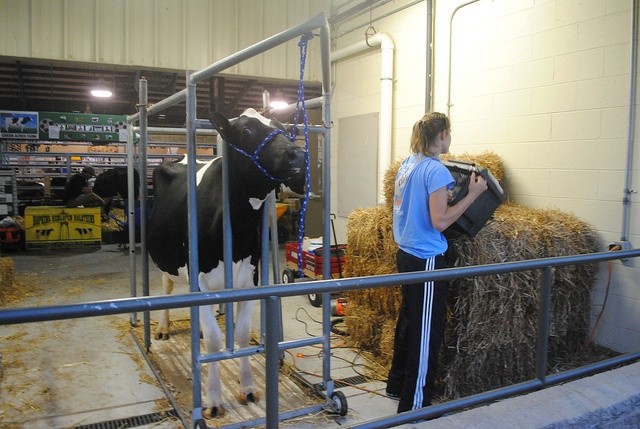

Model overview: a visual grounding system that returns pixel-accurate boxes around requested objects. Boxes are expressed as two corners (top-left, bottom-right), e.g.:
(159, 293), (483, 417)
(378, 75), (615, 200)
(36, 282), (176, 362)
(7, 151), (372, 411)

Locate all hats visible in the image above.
(83, 166), (96, 175)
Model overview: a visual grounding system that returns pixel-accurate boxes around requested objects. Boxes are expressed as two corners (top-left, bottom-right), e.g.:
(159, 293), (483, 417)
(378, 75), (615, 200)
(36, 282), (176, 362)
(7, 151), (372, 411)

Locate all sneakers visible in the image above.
(387, 391), (401, 400)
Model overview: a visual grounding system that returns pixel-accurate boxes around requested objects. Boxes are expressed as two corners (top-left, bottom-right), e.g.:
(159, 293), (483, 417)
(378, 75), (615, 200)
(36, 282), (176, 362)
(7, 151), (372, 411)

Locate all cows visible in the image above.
(147, 107), (308, 418)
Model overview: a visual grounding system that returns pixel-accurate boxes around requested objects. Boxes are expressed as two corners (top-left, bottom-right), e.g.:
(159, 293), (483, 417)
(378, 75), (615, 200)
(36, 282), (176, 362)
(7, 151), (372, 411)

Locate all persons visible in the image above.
(386, 112), (488, 413)
(64, 167), (108, 208)
